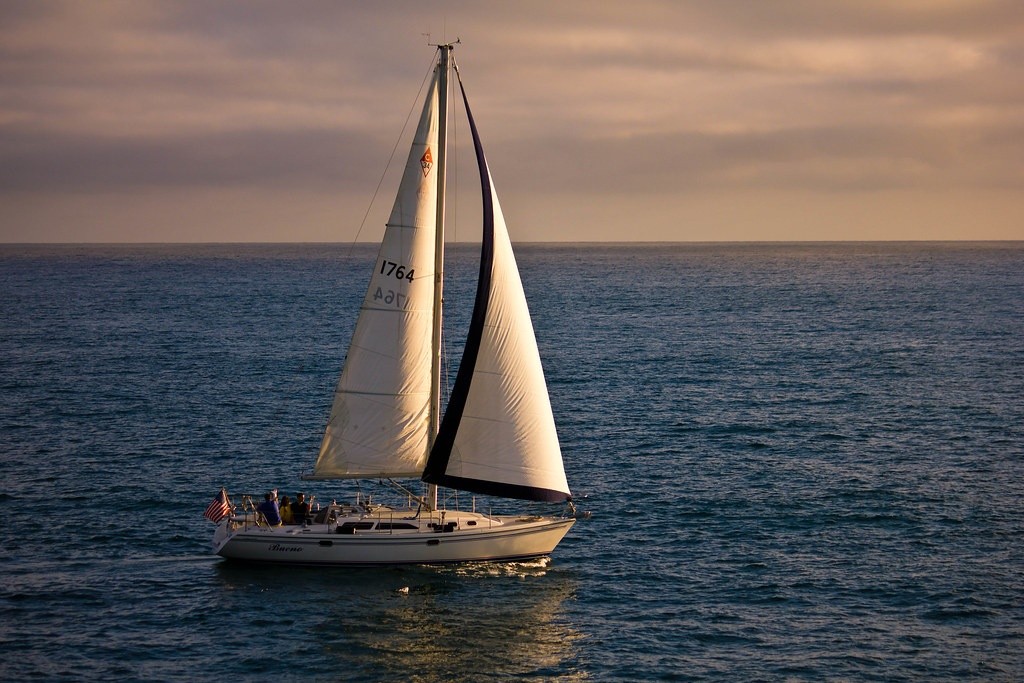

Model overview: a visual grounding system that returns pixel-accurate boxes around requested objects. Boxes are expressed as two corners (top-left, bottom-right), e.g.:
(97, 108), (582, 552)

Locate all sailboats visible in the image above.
(209, 32), (577, 565)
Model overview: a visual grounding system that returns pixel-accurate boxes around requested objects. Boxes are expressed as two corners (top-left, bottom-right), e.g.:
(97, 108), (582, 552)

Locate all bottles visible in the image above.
(316, 502), (320, 511)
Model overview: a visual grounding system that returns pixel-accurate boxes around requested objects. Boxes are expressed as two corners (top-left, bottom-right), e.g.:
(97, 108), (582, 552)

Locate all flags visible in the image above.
(203, 489), (232, 524)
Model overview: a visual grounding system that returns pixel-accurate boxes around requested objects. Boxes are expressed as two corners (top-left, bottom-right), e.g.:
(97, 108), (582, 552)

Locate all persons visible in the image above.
(257, 494), (282, 527)
(290, 493), (309, 524)
(279, 496), (292, 523)
